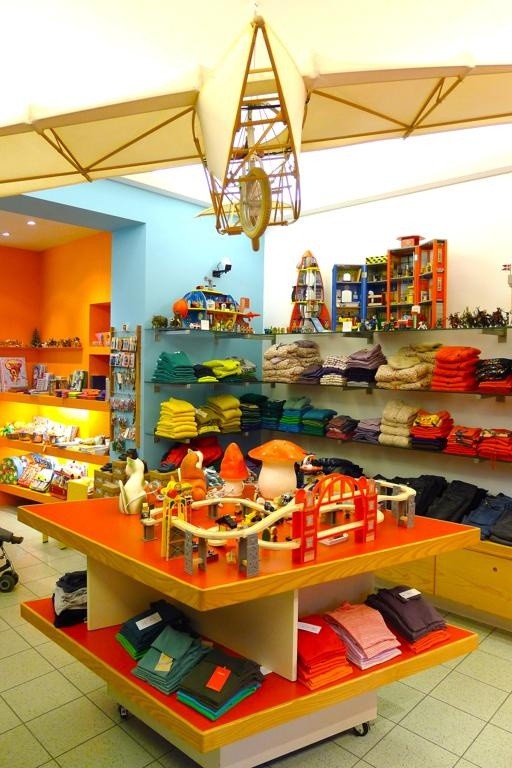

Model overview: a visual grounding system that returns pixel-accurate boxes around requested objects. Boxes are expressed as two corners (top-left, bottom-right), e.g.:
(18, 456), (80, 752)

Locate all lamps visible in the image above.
(213, 257), (232, 278)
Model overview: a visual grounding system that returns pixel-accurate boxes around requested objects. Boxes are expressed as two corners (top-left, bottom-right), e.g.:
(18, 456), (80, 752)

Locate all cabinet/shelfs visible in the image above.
(1, 324), (511, 768)
